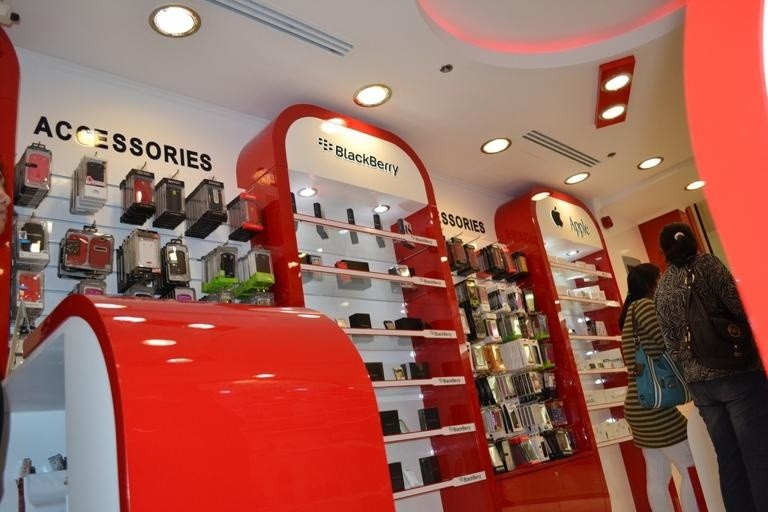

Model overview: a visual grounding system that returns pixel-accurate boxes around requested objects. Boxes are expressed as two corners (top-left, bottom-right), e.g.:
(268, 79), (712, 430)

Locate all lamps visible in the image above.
(299, 186), (317, 198)
(480, 138), (511, 155)
(600, 105), (625, 120)
(353, 85), (392, 108)
(686, 180), (704, 190)
(638, 157), (663, 171)
(601, 74), (631, 92)
(568, 249), (579, 258)
(563, 171), (589, 185)
(372, 205), (390, 215)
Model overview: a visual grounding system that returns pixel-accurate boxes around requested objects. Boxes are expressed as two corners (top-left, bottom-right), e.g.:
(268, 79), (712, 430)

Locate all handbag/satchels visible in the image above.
(636, 337), (695, 410)
(684, 281), (759, 371)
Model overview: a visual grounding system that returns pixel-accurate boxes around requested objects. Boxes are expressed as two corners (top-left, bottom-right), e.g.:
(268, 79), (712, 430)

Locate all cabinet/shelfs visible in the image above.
(495, 188), (708, 512)
(444, 227), (607, 510)
(240, 104), (494, 511)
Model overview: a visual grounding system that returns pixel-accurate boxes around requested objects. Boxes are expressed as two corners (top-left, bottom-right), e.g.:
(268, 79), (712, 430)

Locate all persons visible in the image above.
(652, 220), (767, 512)
(618, 260), (705, 511)
